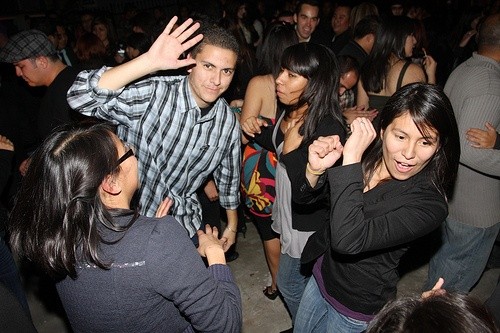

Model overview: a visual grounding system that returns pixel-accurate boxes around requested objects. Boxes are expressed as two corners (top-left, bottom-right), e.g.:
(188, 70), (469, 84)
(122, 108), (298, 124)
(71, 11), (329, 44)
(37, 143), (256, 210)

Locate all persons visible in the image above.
(292, 82), (462, 333)
(7, 119), (243, 333)
(0, 0), (500, 333)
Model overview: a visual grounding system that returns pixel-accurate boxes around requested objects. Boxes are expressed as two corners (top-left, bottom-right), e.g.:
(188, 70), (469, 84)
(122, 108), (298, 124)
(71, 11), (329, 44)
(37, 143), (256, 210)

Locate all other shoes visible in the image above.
(225, 251), (239, 262)
(280, 328), (293, 333)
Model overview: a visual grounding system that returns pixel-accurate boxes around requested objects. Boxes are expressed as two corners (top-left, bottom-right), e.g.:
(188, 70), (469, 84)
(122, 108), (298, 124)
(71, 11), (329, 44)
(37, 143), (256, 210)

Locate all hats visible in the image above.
(0, 30), (54, 63)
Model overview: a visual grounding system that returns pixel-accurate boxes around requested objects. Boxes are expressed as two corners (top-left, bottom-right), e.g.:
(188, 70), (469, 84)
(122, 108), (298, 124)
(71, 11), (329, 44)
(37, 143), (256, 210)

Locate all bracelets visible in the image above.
(305, 161), (326, 176)
(226, 226), (237, 233)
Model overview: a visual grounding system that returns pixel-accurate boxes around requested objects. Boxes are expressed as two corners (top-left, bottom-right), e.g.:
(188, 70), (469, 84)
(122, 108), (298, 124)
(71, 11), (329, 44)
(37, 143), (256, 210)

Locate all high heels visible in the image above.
(237, 223), (247, 238)
(263, 286), (282, 300)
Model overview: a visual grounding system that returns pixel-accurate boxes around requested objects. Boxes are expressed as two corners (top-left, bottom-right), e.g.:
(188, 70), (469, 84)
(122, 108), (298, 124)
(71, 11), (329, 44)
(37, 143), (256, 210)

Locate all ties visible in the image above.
(58, 52), (68, 66)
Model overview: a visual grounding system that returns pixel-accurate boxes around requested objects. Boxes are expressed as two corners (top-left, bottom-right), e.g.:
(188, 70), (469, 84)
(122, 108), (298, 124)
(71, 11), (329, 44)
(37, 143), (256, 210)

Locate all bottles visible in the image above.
(116, 43), (125, 57)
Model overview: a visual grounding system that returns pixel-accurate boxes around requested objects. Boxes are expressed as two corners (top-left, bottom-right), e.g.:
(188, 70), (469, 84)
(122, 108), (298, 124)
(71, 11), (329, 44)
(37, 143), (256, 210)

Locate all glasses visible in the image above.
(105, 141), (136, 176)
